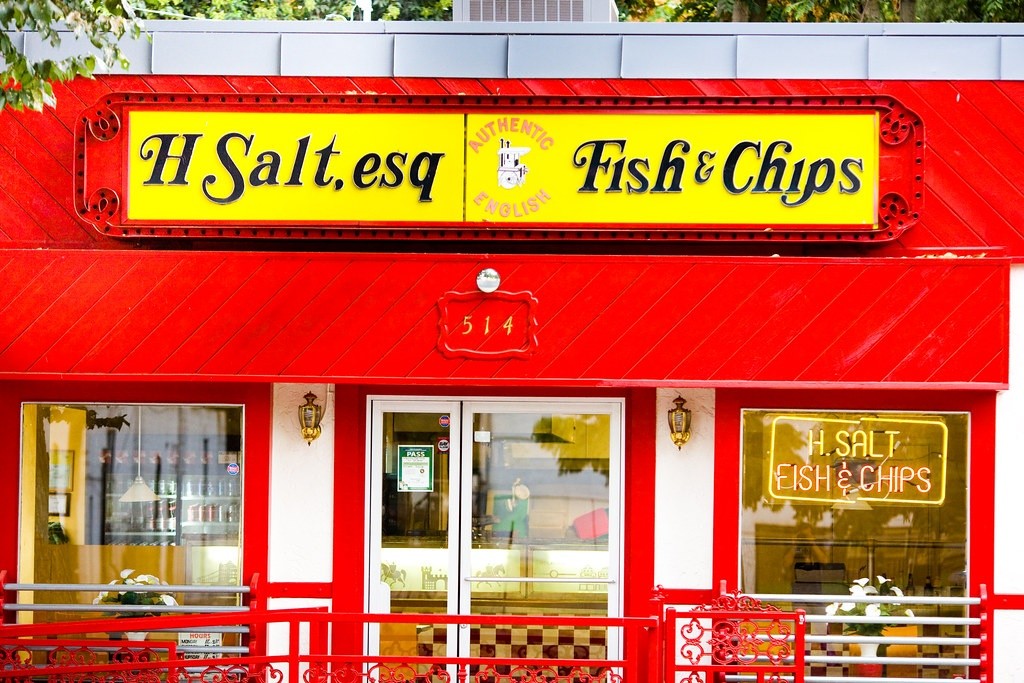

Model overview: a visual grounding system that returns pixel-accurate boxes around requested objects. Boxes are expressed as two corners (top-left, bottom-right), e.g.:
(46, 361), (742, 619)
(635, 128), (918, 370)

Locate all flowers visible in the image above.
(92, 568), (179, 619)
(824, 576), (914, 637)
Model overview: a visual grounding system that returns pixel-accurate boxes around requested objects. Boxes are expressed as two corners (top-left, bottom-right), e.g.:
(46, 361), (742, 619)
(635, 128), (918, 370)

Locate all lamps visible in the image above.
(118, 406), (161, 503)
(668, 395), (691, 451)
(298, 391), (321, 445)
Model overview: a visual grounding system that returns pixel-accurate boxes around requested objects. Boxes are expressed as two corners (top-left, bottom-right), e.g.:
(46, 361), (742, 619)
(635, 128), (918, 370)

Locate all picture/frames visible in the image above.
(49, 449), (74, 492)
(47, 492), (71, 517)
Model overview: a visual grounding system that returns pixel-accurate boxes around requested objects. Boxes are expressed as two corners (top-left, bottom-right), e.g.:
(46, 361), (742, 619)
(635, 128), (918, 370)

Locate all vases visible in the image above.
(856, 644), (883, 677)
(121, 632), (150, 641)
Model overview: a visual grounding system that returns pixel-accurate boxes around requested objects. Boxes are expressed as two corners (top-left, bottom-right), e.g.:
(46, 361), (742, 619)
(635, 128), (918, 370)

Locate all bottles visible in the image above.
(879, 573), (889, 595)
(905, 573), (915, 596)
(890, 577), (897, 596)
(924, 576), (933, 596)
